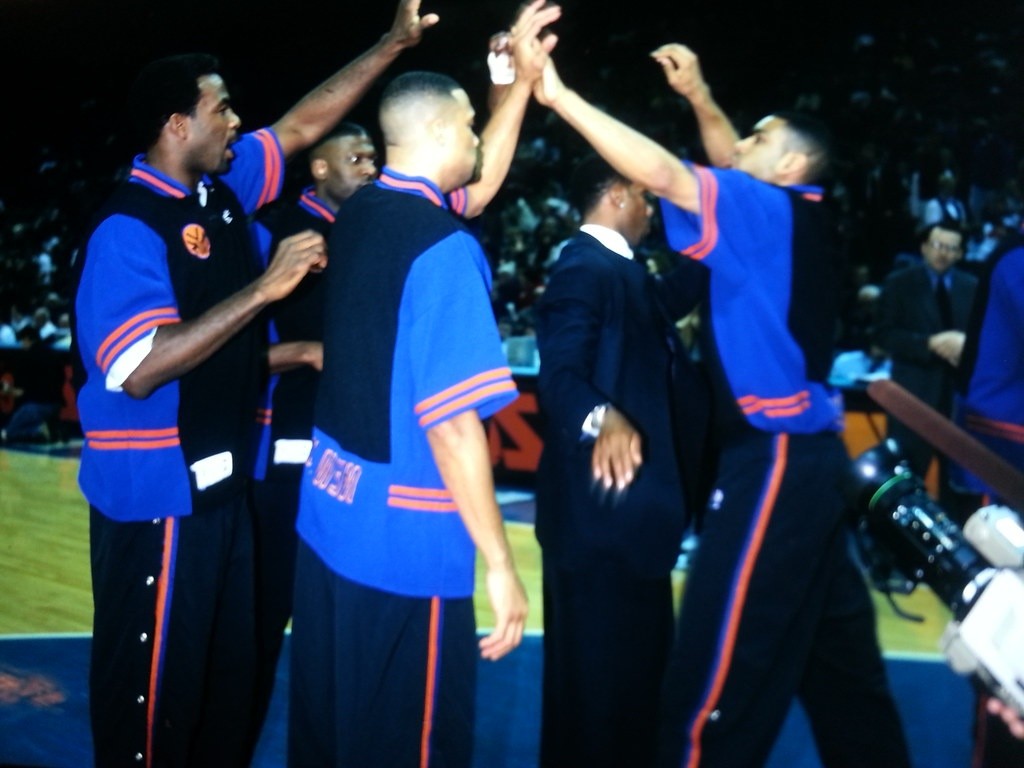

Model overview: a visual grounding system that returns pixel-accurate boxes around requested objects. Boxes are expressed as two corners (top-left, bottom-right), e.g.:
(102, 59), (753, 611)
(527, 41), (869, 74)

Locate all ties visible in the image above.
(936, 276), (954, 331)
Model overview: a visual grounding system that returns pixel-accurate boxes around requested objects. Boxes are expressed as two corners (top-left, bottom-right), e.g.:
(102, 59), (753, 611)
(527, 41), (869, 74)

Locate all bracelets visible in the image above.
(590, 406), (608, 429)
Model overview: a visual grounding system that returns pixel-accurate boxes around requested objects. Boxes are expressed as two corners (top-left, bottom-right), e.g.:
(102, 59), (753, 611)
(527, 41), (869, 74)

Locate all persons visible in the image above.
(247, 124), (378, 768)
(489, 28), (913, 768)
(1, 0), (1024, 392)
(524, 153), (692, 768)
(286, 0), (562, 768)
(68, 0), (439, 768)
(947, 246), (1024, 515)
(871, 222), (980, 501)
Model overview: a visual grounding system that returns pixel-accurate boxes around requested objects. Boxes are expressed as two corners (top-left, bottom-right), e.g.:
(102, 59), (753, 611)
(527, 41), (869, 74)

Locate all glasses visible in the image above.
(929, 241), (960, 253)
